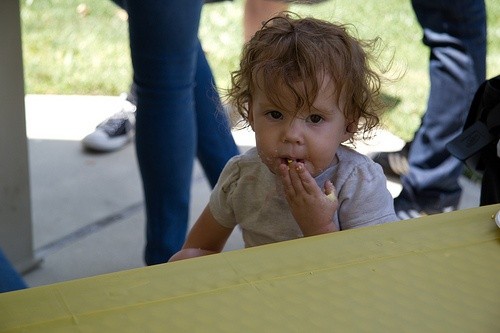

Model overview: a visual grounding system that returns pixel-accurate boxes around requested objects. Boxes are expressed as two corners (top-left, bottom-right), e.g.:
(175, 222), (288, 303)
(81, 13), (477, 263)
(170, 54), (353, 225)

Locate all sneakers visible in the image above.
(83, 112), (136, 152)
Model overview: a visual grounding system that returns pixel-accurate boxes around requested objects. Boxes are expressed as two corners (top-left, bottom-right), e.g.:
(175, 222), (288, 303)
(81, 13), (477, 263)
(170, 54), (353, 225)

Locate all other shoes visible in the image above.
(367, 141), (413, 185)
(391, 189), (462, 222)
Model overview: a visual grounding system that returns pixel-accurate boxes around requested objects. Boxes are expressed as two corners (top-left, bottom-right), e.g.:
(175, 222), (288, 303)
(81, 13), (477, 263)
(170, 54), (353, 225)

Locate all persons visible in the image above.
(223, 3), (328, 129)
(456, 73), (500, 206)
(112, 1), (243, 270)
(79, 78), (138, 156)
(165, 10), (403, 265)
(363, 0), (488, 222)
